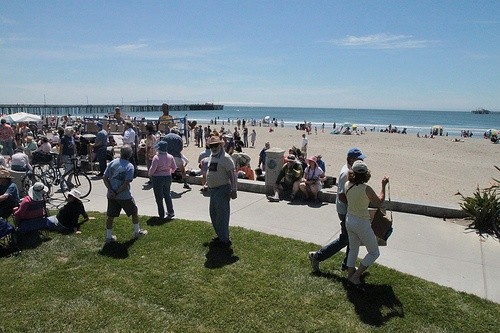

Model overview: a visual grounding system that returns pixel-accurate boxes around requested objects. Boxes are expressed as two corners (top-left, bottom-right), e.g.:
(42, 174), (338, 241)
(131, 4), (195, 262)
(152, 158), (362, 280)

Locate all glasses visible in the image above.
(288, 149), (293, 152)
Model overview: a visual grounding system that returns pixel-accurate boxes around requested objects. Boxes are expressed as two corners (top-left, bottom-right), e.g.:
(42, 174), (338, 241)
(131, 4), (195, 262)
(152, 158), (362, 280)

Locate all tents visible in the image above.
(0, 111), (43, 123)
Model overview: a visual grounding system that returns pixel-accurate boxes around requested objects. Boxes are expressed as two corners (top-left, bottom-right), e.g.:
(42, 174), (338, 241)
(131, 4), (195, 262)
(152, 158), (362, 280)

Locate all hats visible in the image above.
(25, 135), (34, 142)
(347, 148), (367, 160)
(284, 154), (297, 163)
(351, 159), (369, 173)
(292, 145), (297, 153)
(28, 181), (50, 202)
(154, 141), (169, 153)
(67, 189), (83, 201)
(306, 156), (318, 166)
(205, 136), (225, 147)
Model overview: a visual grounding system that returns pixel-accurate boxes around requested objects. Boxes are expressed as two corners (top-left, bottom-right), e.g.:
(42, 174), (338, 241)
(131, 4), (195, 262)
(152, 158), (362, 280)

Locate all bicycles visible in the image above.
(22, 151), (92, 199)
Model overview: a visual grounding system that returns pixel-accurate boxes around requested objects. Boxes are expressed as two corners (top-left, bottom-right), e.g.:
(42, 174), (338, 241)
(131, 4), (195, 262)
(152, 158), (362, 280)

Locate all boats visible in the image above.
(471, 107), (490, 114)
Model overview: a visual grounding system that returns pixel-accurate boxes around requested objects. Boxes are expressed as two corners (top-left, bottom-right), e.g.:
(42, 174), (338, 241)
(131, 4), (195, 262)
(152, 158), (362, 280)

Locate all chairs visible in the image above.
(12, 200), (50, 241)
(0, 216), (22, 259)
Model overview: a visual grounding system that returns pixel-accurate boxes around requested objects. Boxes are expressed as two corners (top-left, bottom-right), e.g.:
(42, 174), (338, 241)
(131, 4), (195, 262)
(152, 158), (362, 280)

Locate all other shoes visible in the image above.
(166, 211), (175, 217)
(183, 183), (191, 190)
(104, 235), (117, 243)
(133, 229), (148, 239)
(346, 279), (371, 298)
(309, 250), (323, 275)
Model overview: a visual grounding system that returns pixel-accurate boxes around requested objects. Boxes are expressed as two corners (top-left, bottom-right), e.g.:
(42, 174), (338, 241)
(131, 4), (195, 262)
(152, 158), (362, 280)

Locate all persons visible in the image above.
(300, 133), (308, 156)
(121, 120), (138, 177)
(0, 169), (19, 219)
(146, 141), (177, 218)
(59, 126), (77, 189)
(92, 121), (109, 176)
(102, 145), (148, 242)
(200, 135), (238, 248)
(46, 189), (96, 234)
(341, 159), (389, 294)
(267, 154), (303, 204)
(298, 156), (327, 206)
(162, 127), (192, 190)
(295, 118), (500, 144)
(308, 148), (369, 271)
(14, 181), (50, 230)
(0, 104), (284, 188)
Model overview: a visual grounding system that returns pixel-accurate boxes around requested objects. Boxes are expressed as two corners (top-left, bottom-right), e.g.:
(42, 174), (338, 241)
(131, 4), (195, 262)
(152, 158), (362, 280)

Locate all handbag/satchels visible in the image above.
(372, 209), (393, 241)
